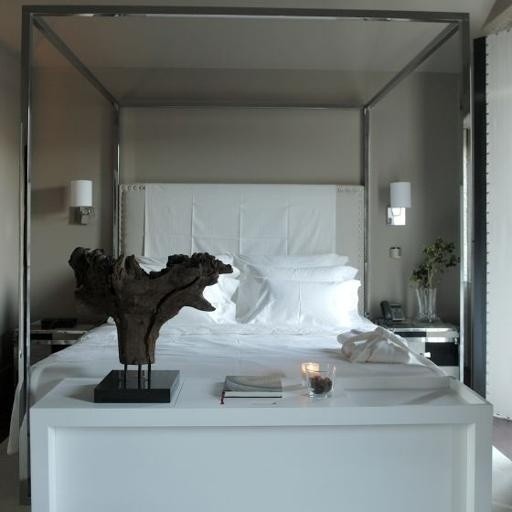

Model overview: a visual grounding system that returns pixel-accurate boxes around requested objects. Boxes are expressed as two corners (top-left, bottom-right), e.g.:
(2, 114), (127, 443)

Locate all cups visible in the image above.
(299, 358), (338, 398)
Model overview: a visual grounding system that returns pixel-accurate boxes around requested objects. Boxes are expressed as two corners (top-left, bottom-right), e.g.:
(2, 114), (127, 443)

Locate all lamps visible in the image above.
(386, 183), (412, 226)
(70, 180), (95, 226)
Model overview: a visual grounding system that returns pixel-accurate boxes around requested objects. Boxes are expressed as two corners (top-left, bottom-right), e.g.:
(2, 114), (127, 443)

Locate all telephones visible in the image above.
(381, 301), (405, 321)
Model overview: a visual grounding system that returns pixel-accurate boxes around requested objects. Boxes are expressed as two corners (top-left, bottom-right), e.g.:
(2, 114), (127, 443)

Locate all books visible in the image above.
(221, 375), (283, 404)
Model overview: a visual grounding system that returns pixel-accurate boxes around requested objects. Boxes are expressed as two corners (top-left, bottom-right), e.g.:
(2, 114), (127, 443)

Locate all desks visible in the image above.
(26, 374), (496, 511)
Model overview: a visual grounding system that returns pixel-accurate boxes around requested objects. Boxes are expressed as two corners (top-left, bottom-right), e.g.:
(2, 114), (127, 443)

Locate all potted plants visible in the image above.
(406, 237), (461, 324)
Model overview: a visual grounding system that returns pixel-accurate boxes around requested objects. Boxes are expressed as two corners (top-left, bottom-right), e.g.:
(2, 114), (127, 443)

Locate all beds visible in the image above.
(5, 182), (450, 507)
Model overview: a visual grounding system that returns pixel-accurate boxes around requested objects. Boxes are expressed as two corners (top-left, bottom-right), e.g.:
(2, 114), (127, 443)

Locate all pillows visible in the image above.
(133, 252), (363, 339)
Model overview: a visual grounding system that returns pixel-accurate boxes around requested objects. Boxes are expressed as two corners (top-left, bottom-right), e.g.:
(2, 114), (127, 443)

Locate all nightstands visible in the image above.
(10, 319), (96, 387)
(378, 319), (460, 380)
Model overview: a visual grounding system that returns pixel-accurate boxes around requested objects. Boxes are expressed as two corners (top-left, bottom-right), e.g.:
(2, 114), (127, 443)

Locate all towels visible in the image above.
(337, 326), (411, 364)
